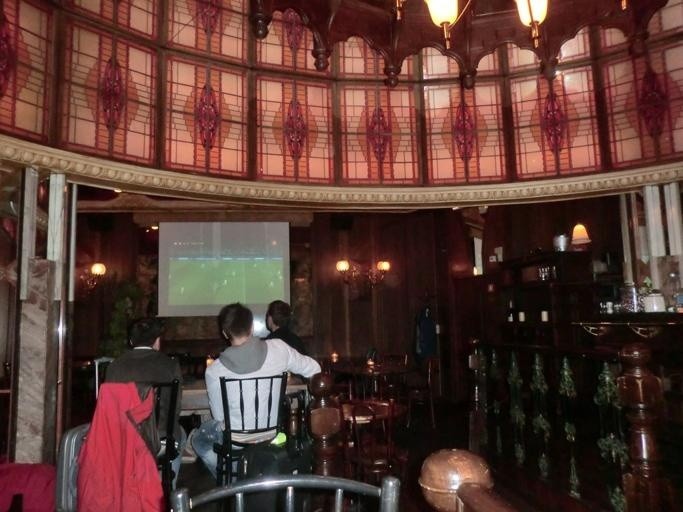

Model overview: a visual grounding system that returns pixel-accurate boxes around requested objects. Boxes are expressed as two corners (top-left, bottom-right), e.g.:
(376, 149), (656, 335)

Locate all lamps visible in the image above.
(333, 255), (390, 289)
(79, 262), (106, 294)
(388, 0), (550, 51)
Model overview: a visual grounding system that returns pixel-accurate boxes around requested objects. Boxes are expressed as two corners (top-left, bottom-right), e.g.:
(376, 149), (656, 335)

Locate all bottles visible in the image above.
(552, 233), (569, 252)
(666, 271), (683, 315)
(506, 299), (514, 322)
(476, 342), (631, 511)
(517, 298), (527, 323)
(540, 292), (550, 324)
(537, 265), (557, 281)
(605, 301), (613, 314)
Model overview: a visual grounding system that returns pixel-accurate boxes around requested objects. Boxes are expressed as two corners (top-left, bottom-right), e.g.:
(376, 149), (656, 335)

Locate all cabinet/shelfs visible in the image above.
(454, 250), (651, 510)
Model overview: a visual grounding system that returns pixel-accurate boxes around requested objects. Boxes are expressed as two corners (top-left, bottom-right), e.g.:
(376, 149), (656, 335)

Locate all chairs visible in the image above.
(338, 351), (435, 473)
(214, 372), (289, 487)
(135, 378), (181, 508)
(168, 474), (401, 510)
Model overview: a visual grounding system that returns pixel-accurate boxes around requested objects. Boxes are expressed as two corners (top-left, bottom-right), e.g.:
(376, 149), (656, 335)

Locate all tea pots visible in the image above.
(638, 289), (666, 312)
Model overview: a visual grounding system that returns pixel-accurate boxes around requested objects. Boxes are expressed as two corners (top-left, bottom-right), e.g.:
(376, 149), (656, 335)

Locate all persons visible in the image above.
(191, 301), (323, 485)
(259, 300), (306, 376)
(104, 318), (188, 491)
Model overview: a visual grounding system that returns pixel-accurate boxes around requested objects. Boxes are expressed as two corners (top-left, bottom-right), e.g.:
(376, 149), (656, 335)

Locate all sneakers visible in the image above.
(179, 443), (198, 465)
